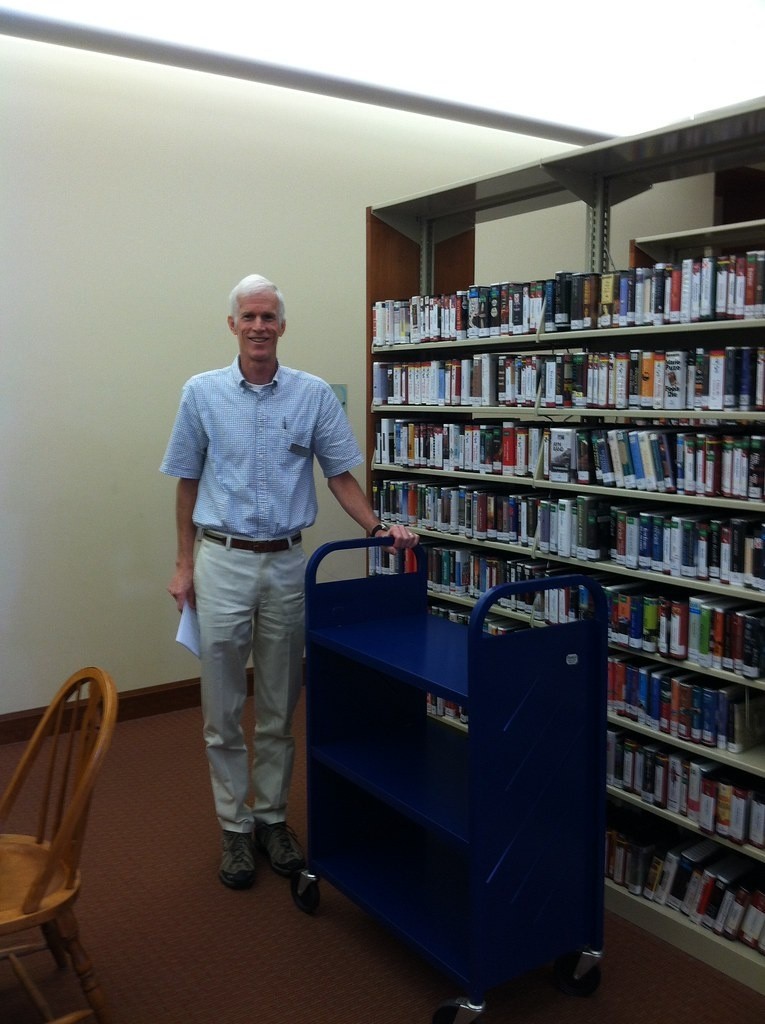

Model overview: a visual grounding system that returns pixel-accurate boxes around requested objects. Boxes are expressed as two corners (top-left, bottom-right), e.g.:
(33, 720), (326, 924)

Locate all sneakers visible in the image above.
(254, 820), (305, 876)
(218, 826), (256, 888)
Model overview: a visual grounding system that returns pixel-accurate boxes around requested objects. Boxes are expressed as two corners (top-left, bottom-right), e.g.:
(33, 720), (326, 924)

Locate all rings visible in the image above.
(408, 532), (414, 536)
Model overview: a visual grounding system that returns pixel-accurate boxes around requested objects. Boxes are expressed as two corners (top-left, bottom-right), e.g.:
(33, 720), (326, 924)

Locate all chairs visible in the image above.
(0, 666), (118, 1024)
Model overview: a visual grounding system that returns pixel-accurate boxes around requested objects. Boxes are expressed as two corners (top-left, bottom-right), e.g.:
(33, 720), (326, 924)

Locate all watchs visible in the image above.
(370, 522), (390, 537)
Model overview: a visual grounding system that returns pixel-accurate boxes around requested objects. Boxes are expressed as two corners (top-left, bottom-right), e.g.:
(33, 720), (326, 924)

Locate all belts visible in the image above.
(202, 529), (301, 553)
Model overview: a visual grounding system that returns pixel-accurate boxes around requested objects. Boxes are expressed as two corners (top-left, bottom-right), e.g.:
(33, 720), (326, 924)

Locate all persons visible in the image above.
(159, 274), (419, 889)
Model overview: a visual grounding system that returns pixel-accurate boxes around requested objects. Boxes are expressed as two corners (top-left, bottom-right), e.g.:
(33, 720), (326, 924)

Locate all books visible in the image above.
(369, 251), (765, 955)
(176, 599), (201, 659)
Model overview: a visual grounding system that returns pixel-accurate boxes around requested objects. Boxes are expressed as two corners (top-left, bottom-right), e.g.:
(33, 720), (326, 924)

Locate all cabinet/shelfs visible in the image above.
(288, 94), (765, 1024)
